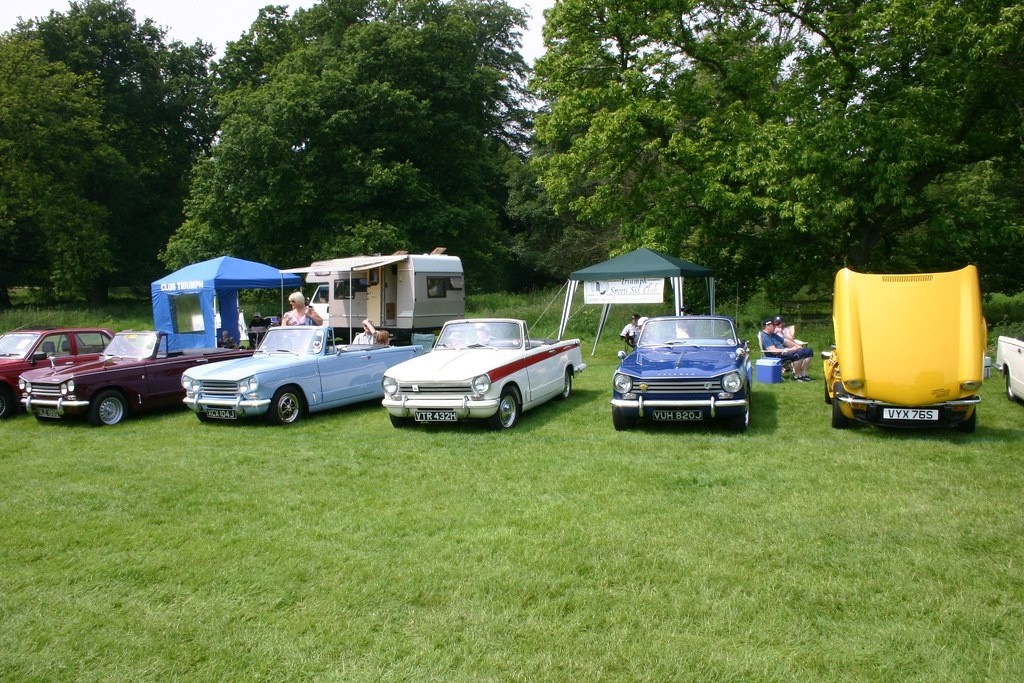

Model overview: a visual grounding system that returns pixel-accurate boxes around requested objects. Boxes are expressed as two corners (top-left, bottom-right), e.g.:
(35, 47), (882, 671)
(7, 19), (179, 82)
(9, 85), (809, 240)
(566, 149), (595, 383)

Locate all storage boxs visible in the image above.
(756, 357), (782, 384)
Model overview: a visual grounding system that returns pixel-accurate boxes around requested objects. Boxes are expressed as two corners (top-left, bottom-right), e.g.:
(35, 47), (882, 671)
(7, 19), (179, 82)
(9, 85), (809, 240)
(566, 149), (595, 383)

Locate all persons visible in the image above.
(272, 313), (282, 326)
(684, 311), (696, 337)
(220, 331), (237, 349)
(249, 312), (268, 347)
(620, 314), (655, 352)
(761, 316), (816, 382)
(282, 292), (323, 326)
(320, 296), (327, 303)
(376, 331), (390, 346)
(353, 319), (378, 345)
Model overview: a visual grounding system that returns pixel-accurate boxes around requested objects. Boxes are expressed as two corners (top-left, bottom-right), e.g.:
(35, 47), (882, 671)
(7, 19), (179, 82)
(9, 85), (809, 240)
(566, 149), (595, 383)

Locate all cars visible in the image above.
(16, 332), (258, 427)
(381, 318), (586, 430)
(0, 327), (141, 422)
(992, 335), (1024, 401)
(820, 265), (986, 432)
(180, 326), (423, 426)
(610, 314), (751, 428)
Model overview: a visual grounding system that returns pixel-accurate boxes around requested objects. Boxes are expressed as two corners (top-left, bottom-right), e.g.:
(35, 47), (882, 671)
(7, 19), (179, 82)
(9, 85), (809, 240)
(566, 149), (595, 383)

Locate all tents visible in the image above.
(558, 247), (716, 356)
(151, 256), (303, 352)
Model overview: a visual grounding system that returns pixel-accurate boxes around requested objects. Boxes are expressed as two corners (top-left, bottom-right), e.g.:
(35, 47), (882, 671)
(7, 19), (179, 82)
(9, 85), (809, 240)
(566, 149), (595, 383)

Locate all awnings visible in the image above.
(279, 255), (406, 344)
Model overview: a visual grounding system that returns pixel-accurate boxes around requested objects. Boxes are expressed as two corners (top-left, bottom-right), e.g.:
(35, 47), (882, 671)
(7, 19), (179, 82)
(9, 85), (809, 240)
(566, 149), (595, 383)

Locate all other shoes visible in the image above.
(793, 375), (803, 382)
(802, 374), (815, 382)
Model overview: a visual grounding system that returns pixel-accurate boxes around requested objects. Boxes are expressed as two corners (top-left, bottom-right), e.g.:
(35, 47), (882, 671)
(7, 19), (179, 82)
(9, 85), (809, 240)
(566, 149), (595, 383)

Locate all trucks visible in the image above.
(305, 255), (465, 343)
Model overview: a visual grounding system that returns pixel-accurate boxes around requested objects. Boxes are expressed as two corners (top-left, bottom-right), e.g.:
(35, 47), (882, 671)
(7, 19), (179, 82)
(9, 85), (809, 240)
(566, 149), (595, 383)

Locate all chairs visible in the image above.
(411, 333), (435, 353)
(757, 332), (802, 379)
(794, 339), (808, 349)
(62, 341), (70, 351)
(43, 342), (55, 356)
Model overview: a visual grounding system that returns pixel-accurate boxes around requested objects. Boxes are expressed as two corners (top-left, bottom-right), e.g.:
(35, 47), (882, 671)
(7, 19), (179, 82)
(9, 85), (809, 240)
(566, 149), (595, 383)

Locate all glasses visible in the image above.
(364, 325), (367, 327)
(631, 317), (634, 319)
(288, 301), (294, 303)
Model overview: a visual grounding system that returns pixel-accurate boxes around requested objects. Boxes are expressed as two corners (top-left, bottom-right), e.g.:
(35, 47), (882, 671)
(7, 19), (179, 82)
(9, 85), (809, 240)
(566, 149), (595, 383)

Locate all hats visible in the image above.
(223, 331), (229, 335)
(763, 318), (775, 325)
(254, 312), (261, 317)
(774, 316), (782, 322)
